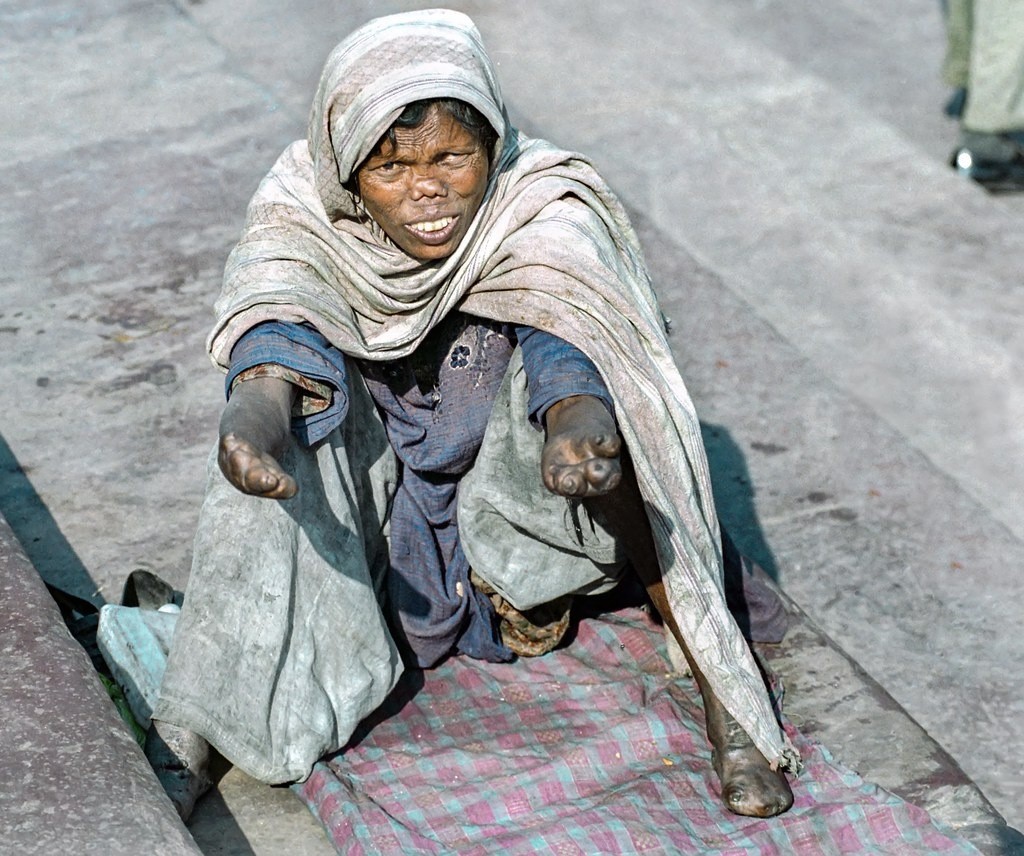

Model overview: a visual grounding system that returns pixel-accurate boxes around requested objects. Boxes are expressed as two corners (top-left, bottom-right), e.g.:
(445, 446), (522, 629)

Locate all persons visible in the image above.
(208, 7), (794, 818)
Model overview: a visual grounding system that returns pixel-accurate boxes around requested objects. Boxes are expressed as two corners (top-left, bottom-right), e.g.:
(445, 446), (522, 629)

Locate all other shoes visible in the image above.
(945, 142), (1024, 194)
